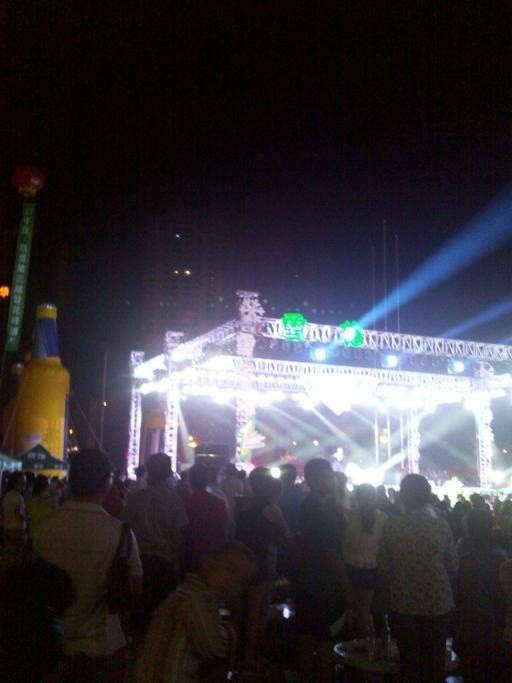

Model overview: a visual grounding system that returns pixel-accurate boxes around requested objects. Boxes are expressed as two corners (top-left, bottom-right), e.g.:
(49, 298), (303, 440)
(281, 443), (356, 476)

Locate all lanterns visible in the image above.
(12, 162), (47, 198)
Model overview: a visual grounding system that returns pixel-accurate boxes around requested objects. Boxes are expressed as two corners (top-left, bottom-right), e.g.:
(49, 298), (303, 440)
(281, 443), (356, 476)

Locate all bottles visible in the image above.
(0, 349), (31, 461)
(378, 611), (392, 654)
(16, 301), (71, 485)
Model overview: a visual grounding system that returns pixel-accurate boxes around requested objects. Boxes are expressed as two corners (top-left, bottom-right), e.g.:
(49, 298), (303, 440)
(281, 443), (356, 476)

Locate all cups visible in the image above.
(445, 639), (455, 663)
